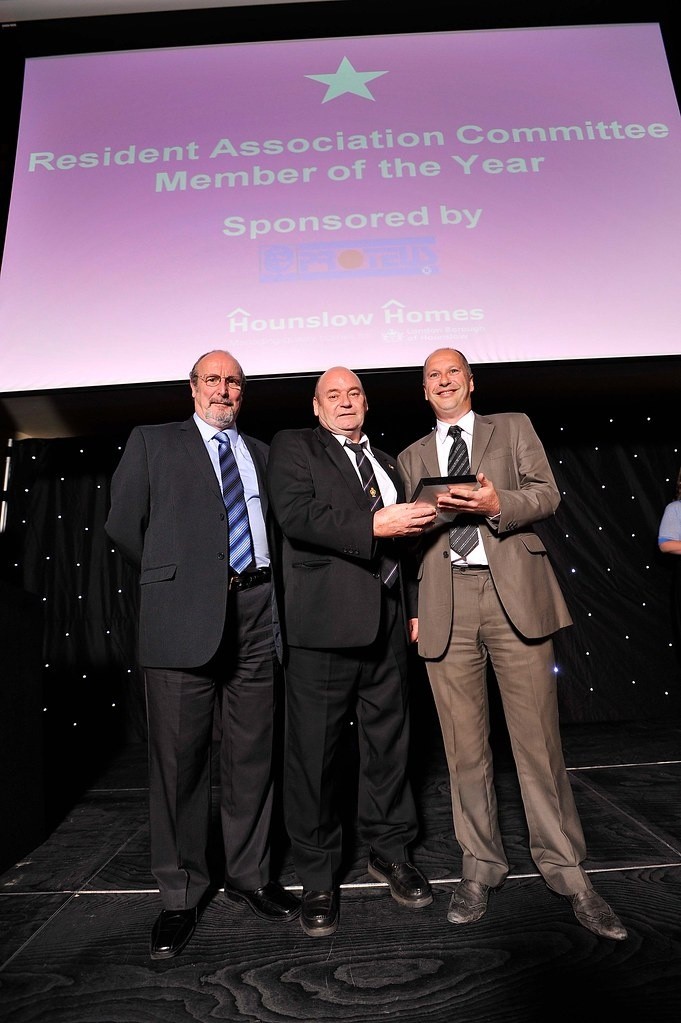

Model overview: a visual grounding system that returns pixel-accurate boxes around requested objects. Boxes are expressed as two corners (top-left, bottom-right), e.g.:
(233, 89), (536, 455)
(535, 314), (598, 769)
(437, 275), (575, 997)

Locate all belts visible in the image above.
(227, 571), (271, 593)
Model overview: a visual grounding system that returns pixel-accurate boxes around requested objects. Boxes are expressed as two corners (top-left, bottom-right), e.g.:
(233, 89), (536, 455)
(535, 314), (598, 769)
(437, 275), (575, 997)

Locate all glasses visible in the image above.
(196, 371), (243, 389)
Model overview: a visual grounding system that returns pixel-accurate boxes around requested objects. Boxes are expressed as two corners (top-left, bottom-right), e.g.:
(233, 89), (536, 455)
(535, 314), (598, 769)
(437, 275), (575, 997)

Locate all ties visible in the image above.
(214, 430), (255, 576)
(345, 440), (399, 589)
(447, 425), (479, 559)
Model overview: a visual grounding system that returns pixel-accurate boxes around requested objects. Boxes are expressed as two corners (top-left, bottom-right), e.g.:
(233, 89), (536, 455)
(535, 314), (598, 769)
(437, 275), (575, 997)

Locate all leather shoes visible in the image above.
(149, 883), (217, 960)
(367, 846), (434, 908)
(446, 877), (491, 924)
(224, 876), (304, 922)
(300, 874), (340, 936)
(566, 887), (628, 941)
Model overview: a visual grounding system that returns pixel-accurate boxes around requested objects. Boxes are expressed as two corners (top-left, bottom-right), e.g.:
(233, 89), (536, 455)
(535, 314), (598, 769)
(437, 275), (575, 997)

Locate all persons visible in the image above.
(267, 365), (439, 942)
(657, 469), (681, 556)
(396, 344), (631, 943)
(101, 349), (303, 962)
